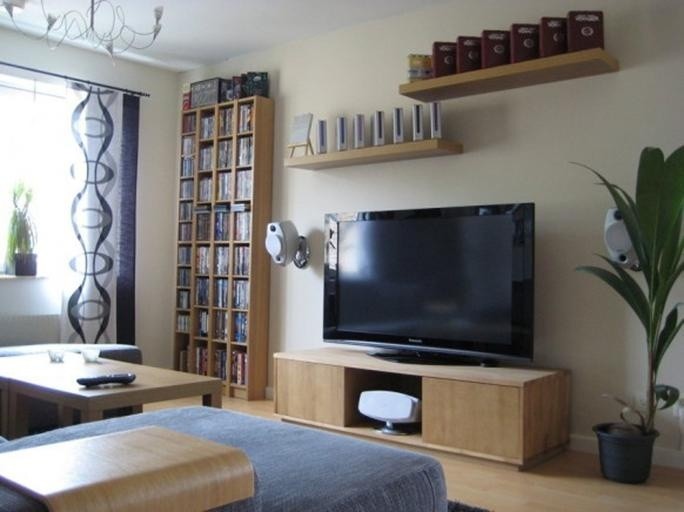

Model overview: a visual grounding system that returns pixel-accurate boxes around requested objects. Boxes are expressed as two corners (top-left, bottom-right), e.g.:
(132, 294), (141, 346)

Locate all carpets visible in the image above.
(447, 499), (491, 512)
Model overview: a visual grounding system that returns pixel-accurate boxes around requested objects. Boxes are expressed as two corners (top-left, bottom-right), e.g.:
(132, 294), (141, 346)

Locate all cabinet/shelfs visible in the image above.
(271, 347), (573, 472)
(282, 47), (620, 172)
(172, 95), (275, 401)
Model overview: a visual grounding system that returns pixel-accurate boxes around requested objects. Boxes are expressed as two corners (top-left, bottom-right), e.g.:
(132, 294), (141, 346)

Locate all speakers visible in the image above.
(357, 390), (421, 435)
(265, 219), (299, 267)
(604, 206), (638, 269)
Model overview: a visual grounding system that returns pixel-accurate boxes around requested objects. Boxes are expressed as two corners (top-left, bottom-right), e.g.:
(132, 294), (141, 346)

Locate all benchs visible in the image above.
(0, 343), (143, 436)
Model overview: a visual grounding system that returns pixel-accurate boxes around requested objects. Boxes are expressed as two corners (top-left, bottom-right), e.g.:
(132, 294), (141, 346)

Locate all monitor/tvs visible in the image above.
(321, 202), (535, 368)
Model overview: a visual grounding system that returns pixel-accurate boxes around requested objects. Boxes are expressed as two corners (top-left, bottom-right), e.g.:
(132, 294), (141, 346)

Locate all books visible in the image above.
(431, 11), (602, 78)
(174, 72), (268, 384)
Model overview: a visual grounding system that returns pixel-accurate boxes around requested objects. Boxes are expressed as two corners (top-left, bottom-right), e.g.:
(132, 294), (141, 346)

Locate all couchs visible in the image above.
(0, 406), (449, 512)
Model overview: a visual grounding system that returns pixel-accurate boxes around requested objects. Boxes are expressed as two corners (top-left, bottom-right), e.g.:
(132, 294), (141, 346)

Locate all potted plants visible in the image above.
(4, 181), (37, 275)
(569, 142), (684, 485)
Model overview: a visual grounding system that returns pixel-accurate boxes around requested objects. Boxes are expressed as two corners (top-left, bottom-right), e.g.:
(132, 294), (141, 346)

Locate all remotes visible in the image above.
(76, 372), (137, 388)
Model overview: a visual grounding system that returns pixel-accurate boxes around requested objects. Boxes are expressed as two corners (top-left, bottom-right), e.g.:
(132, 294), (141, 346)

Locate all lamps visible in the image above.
(265, 220), (310, 269)
(0, 0), (164, 68)
(602, 205), (642, 273)
